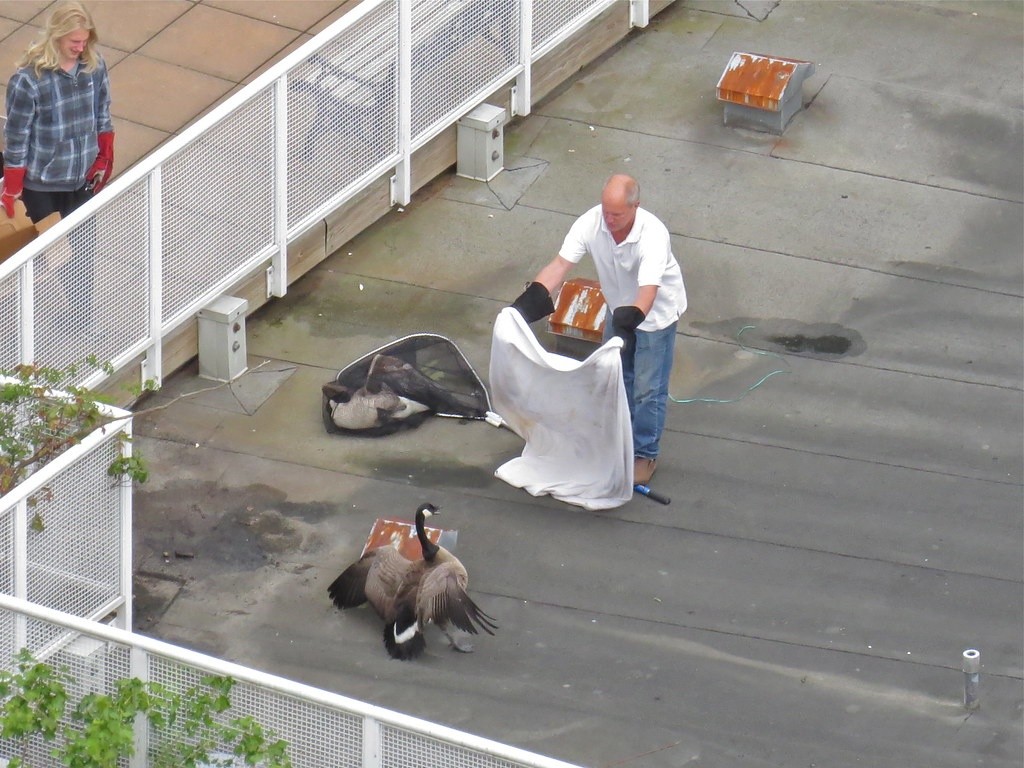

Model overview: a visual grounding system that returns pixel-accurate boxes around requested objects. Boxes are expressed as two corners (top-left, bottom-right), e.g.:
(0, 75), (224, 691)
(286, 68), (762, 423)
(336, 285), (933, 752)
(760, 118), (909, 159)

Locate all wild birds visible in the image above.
(327, 502), (499, 661)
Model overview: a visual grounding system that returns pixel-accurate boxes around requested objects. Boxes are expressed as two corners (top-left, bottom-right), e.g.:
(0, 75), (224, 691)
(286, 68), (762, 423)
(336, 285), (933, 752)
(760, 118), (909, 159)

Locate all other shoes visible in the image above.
(634, 457), (658, 485)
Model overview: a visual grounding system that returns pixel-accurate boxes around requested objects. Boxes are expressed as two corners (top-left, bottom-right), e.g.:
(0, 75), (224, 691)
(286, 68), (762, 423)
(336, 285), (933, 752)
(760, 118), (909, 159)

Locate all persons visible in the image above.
(510, 174), (688, 486)
(0, 0), (115, 342)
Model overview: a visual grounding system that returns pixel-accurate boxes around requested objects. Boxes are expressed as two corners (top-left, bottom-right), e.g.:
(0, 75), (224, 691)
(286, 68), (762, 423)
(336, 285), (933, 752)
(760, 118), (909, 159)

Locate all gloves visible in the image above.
(87, 132), (115, 194)
(0, 167), (25, 218)
(510, 282), (554, 324)
(609, 306), (646, 356)
